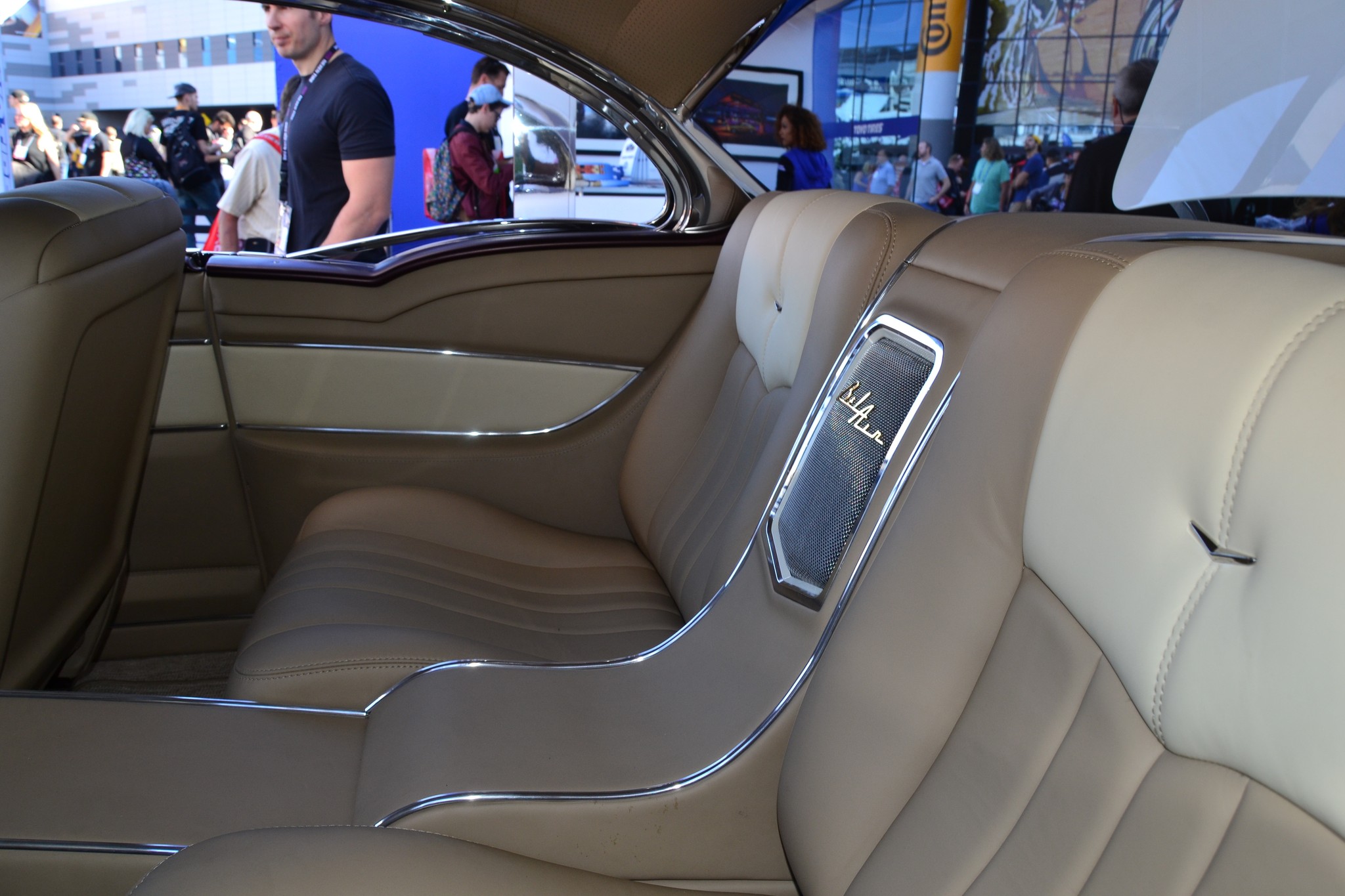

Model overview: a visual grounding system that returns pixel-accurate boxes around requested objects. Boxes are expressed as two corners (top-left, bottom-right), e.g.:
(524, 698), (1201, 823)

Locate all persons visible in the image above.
(262, 4), (394, 266)
(443, 56), (513, 219)
(158, 82), (222, 247)
(1065, 57), (1233, 225)
(775, 105), (833, 190)
(428, 83), (514, 221)
(836, 122), (1345, 239)
(7, 79), (278, 189)
(215, 75), (307, 251)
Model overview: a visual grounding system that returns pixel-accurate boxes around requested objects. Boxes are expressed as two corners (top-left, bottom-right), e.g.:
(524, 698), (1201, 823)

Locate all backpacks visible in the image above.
(164, 110), (215, 189)
(425, 127), (486, 221)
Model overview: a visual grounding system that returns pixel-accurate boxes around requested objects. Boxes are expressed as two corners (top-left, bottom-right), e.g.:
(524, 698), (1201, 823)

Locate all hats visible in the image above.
(168, 83), (196, 98)
(11, 89), (30, 103)
(76, 112), (98, 122)
(467, 84), (512, 108)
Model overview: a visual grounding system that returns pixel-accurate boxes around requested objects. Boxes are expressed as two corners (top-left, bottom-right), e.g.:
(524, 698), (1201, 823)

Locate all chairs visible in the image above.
(126, 188), (1344, 894)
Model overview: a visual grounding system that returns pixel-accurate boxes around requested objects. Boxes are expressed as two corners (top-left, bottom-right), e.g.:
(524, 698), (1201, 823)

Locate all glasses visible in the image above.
(490, 106), (501, 122)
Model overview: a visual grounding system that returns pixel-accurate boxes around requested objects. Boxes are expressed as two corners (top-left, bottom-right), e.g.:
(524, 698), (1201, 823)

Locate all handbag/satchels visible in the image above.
(124, 138), (160, 178)
